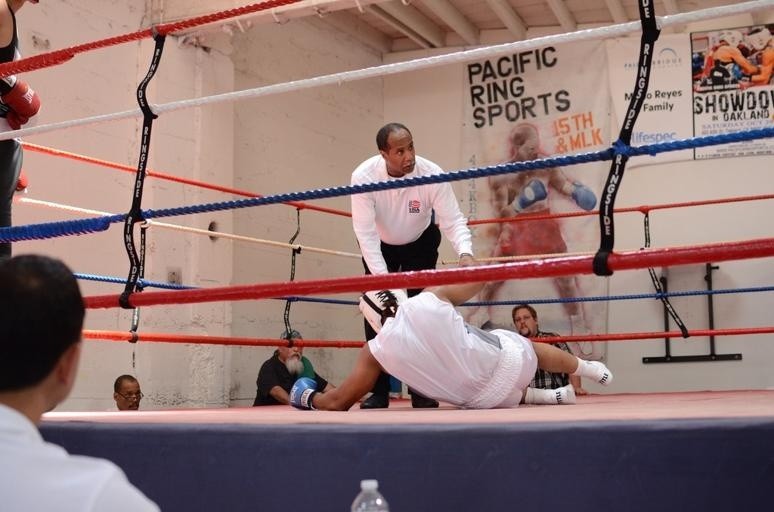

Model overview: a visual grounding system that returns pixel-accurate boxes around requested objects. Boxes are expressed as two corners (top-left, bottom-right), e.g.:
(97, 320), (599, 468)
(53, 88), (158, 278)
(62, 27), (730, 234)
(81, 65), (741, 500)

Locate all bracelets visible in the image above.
(460, 253), (471, 258)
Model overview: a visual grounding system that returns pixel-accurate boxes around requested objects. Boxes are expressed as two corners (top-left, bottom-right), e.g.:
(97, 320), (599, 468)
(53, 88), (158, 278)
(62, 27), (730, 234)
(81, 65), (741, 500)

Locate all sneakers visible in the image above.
(590, 360), (612, 387)
(546, 383), (576, 405)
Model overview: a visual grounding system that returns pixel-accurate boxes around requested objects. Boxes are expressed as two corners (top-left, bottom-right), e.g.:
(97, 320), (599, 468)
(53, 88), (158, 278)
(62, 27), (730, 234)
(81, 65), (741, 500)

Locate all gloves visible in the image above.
(571, 182), (596, 210)
(514, 181), (546, 212)
(0, 76), (41, 130)
(289, 377), (319, 409)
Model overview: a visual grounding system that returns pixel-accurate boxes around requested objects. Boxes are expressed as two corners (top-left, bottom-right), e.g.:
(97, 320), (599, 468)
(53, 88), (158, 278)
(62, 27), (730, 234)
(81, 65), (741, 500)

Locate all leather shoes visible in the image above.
(361, 393), (389, 408)
(411, 396), (439, 408)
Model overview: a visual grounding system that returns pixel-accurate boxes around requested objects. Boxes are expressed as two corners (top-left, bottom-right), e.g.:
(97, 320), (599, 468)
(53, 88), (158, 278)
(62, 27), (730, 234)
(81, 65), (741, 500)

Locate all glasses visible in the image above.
(115, 391), (144, 403)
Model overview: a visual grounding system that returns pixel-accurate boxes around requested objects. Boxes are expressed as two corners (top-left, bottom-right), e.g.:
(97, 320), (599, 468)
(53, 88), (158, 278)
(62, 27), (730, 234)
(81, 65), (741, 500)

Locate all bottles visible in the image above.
(351, 477), (387, 511)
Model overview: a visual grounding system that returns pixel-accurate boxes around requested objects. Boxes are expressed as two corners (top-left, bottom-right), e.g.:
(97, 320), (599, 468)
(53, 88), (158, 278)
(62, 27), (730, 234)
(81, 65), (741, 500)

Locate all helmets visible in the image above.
(749, 28), (771, 51)
(718, 31), (745, 47)
(360, 289), (407, 333)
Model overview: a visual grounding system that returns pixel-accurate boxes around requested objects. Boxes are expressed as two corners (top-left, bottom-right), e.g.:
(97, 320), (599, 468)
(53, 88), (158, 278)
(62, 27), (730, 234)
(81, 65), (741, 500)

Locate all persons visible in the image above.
(477, 123), (601, 357)
(0, 0), (44, 257)
(694, 22), (774, 86)
(1, 251), (166, 512)
(288, 259), (614, 410)
(507, 303), (600, 398)
(111, 374), (145, 410)
(348, 121), (474, 409)
(250, 327), (342, 405)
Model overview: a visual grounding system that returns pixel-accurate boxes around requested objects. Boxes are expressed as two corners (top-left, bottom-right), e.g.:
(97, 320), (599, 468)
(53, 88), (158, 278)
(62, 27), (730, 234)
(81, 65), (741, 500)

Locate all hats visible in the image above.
(279, 330), (301, 340)
